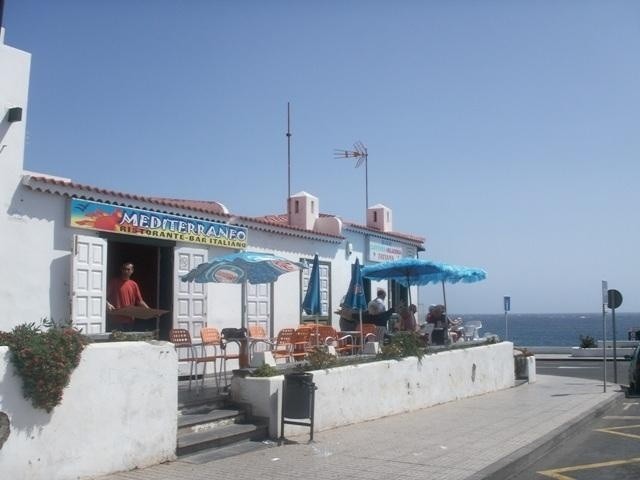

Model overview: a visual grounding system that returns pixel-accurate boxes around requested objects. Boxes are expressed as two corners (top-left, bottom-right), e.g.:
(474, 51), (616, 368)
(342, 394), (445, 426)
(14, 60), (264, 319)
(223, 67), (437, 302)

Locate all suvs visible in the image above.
(623, 344), (640, 394)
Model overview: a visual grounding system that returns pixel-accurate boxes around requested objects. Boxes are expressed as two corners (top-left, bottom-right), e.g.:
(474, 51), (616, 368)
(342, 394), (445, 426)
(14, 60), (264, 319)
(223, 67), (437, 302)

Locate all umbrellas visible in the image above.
(340, 256), (369, 352)
(396, 265), (488, 339)
(181, 249), (311, 364)
(299, 252), (324, 347)
(360, 257), (444, 320)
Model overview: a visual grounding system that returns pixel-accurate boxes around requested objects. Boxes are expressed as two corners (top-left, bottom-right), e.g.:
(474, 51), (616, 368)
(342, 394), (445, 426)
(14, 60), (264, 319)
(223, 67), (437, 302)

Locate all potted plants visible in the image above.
(513, 347), (536, 384)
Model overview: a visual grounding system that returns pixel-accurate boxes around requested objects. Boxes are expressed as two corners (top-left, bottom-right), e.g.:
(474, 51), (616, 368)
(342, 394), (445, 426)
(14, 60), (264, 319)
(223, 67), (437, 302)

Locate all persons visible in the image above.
(106, 261), (152, 331)
(365, 288), (395, 328)
(401, 301), (463, 347)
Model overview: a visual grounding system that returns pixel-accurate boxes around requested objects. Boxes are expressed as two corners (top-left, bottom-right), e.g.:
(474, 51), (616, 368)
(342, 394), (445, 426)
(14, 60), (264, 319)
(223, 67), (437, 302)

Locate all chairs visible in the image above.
(296, 322), (484, 361)
(249, 326), (268, 361)
(269, 328), (295, 367)
(200, 328), (237, 387)
(170, 328), (219, 392)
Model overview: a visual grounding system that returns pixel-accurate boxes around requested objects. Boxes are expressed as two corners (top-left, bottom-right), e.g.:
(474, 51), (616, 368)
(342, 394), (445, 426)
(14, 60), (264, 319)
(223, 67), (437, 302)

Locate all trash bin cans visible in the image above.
(281, 372), (318, 440)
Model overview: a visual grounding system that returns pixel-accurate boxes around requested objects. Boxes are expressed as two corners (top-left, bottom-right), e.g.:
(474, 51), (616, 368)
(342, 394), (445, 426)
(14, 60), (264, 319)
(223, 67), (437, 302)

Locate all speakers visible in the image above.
(346, 242), (352, 255)
(10, 108), (24, 121)
